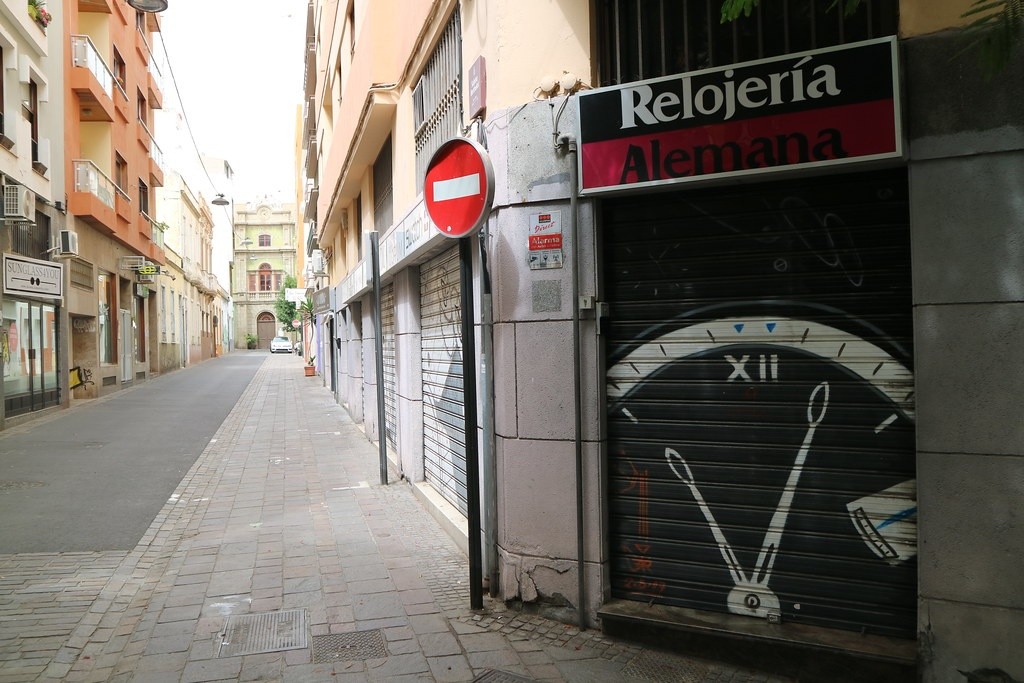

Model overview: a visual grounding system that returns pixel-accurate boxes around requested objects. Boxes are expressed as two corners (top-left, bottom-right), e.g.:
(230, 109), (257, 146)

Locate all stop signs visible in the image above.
(292, 319), (300, 328)
(423, 135), (495, 241)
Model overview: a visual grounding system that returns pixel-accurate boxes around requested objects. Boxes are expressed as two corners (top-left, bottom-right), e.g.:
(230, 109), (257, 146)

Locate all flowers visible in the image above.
(37, 9), (52, 26)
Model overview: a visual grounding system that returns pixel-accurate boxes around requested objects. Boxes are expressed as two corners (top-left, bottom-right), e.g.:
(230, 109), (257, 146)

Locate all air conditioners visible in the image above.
(139, 261), (154, 283)
(3, 185), (36, 222)
(59, 230), (78, 256)
(311, 250), (323, 274)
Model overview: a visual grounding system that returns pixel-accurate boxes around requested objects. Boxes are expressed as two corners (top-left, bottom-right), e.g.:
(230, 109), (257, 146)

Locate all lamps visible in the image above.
(212, 193), (229, 205)
(126, 0), (168, 13)
(241, 237), (254, 245)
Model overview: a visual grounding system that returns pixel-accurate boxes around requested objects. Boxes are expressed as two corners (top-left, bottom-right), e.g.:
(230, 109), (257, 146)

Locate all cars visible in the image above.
(270, 336), (293, 353)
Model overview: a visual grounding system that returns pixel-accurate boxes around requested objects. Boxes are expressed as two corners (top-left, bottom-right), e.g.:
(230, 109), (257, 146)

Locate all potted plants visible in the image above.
(304, 355), (316, 376)
(246, 333), (259, 349)
(28, 0), (46, 21)
